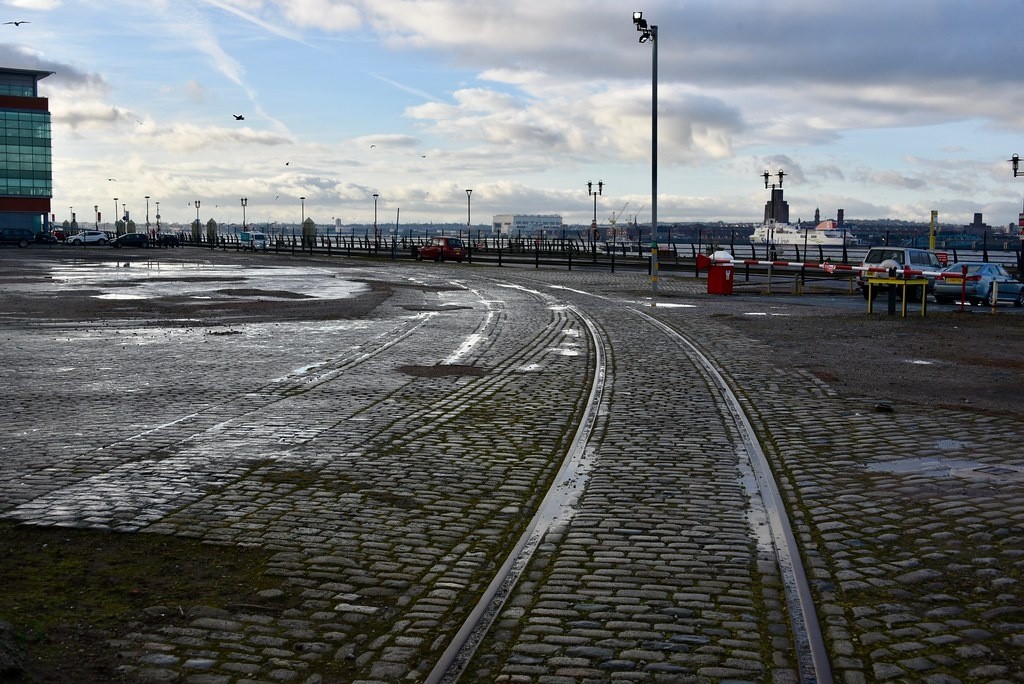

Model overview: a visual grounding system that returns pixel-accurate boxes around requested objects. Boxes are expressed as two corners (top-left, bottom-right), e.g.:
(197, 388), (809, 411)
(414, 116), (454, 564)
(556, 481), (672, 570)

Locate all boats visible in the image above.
(747, 213), (862, 247)
(597, 235), (652, 253)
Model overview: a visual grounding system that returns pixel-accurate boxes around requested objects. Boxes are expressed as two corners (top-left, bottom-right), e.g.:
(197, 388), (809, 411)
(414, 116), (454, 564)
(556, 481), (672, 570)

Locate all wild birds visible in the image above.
(286, 161), (290, 166)
(187, 202), (191, 205)
(215, 204), (218, 208)
(276, 195), (280, 200)
(421, 155), (426, 159)
(233, 114), (245, 121)
(105, 178), (117, 181)
(137, 120), (143, 124)
(4, 21), (31, 27)
(370, 145), (376, 148)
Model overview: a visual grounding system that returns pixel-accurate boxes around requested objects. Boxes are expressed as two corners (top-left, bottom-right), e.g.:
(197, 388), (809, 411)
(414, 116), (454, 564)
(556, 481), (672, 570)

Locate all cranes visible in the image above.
(607, 201), (630, 226)
(625, 205), (645, 224)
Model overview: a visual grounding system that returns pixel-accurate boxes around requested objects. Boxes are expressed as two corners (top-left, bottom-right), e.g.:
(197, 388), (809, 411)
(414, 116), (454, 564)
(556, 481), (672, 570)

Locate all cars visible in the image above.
(35, 231), (58, 245)
(931, 261), (1024, 308)
(108, 232), (150, 249)
(155, 234), (180, 247)
(415, 236), (467, 263)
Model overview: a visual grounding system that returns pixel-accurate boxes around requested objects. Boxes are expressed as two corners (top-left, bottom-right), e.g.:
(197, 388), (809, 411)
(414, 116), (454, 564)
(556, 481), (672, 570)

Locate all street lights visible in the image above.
(155, 202), (160, 238)
(585, 179), (606, 263)
(759, 169), (789, 261)
(194, 200), (201, 245)
(122, 204), (127, 233)
(113, 197), (119, 237)
(94, 205), (98, 231)
(69, 206), (73, 221)
(631, 9), (660, 306)
(466, 189), (473, 264)
(144, 196), (152, 233)
(299, 196), (305, 249)
(240, 198), (248, 232)
(372, 194), (380, 253)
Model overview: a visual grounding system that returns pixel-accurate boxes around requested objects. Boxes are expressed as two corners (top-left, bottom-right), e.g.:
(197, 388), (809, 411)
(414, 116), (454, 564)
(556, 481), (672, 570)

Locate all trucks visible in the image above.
(240, 230), (271, 252)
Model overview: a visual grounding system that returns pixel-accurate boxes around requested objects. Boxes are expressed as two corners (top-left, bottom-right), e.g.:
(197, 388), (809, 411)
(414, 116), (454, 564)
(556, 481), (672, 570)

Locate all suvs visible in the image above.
(0, 226), (35, 248)
(855, 247), (949, 304)
(66, 231), (109, 246)
